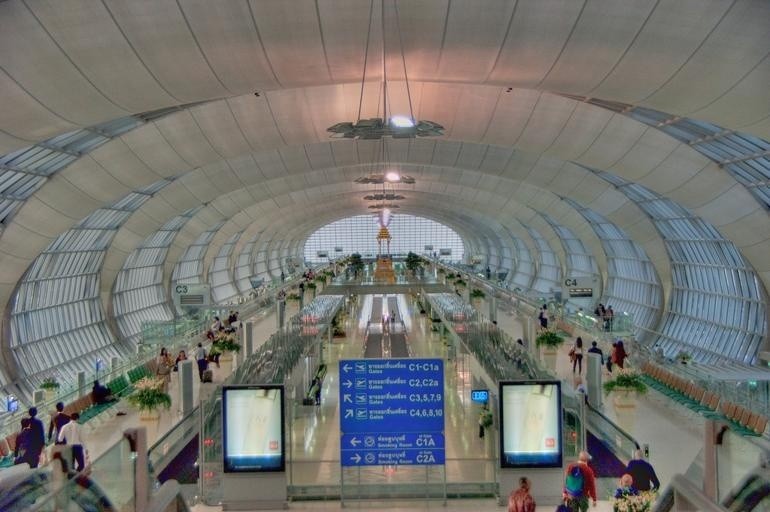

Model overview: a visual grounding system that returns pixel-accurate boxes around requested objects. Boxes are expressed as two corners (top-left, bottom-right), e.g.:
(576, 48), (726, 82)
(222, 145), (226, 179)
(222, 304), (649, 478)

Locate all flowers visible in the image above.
(209, 331), (242, 356)
(534, 326), (565, 350)
(124, 373), (176, 413)
(600, 365), (649, 397)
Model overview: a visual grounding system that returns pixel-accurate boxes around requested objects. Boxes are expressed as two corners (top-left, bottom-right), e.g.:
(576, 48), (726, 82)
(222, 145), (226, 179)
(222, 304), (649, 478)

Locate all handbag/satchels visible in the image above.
(13, 447), (34, 466)
(567, 346), (576, 356)
(84, 449), (92, 476)
(555, 499), (573, 512)
(538, 312), (544, 319)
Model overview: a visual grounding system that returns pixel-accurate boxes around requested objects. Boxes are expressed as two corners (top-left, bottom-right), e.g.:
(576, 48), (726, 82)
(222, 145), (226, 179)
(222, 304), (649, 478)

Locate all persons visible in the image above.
(93, 381), (123, 404)
(315, 376), (322, 405)
(539, 304), (628, 375)
(27, 407), (44, 446)
(155, 311), (237, 393)
(13, 418), (41, 467)
(508, 450), (659, 512)
(48, 402), (70, 443)
(58, 413), (88, 472)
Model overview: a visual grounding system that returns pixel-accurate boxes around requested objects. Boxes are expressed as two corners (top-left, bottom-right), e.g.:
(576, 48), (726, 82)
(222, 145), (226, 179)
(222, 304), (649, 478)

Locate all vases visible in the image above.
(541, 343), (558, 355)
(137, 402), (161, 421)
(611, 384), (638, 408)
(219, 350), (234, 362)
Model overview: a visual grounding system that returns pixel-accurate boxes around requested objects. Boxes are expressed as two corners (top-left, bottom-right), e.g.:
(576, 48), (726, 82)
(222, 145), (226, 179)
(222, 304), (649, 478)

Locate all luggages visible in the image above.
(202, 365), (213, 384)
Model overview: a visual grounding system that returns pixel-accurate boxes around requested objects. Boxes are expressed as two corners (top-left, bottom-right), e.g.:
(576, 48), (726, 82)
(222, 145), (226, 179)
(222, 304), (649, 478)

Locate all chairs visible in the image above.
(637, 360), (769, 438)
(0, 356), (158, 471)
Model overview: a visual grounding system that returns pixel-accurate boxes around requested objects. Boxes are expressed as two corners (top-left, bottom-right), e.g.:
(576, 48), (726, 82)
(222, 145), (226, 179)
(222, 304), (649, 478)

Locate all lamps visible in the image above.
(324, 0), (446, 229)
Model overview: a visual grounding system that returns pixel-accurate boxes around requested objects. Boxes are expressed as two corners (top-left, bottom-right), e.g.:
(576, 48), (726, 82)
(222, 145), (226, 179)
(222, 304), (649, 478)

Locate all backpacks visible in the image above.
(565, 464), (585, 498)
